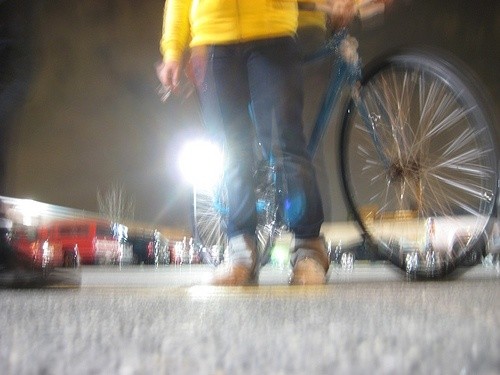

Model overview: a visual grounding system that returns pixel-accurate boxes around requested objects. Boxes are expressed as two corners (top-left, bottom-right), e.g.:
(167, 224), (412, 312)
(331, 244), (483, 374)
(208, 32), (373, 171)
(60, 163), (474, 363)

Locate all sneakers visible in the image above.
(210, 235), (262, 285)
(287, 237), (332, 283)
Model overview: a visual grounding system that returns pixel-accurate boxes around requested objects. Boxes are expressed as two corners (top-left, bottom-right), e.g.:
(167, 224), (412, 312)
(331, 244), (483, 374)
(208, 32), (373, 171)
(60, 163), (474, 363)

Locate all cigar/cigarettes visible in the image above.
(160, 82), (175, 105)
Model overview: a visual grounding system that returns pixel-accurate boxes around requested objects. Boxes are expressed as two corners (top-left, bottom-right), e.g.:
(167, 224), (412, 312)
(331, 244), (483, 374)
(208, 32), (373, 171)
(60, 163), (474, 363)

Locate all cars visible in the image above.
(9, 215), (499, 274)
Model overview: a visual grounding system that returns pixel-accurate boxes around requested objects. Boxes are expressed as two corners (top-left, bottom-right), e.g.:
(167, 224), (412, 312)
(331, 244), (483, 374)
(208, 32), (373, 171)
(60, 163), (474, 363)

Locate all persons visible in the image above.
(0, 1), (83, 291)
(157, 0), (391, 287)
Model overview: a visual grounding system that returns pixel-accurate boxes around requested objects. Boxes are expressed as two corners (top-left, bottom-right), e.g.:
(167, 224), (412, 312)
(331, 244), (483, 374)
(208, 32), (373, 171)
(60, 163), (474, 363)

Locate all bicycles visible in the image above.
(190, 0), (498, 282)
